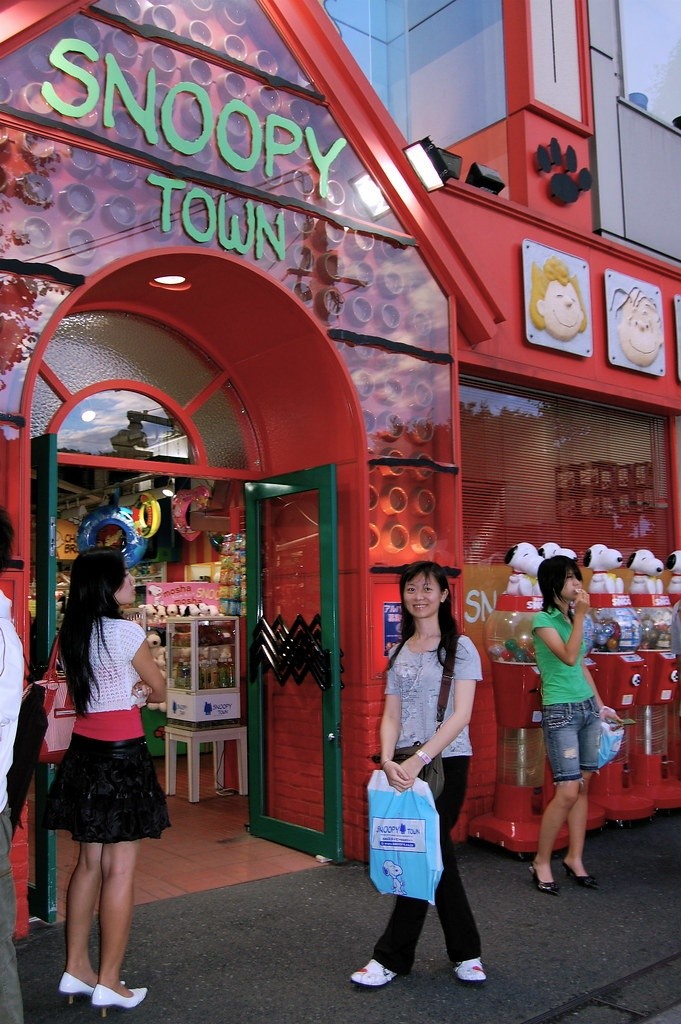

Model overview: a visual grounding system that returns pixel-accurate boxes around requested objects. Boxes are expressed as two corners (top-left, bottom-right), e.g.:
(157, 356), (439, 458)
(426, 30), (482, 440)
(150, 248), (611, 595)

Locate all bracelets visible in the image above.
(382, 759), (389, 767)
(599, 705), (609, 714)
(415, 750), (431, 764)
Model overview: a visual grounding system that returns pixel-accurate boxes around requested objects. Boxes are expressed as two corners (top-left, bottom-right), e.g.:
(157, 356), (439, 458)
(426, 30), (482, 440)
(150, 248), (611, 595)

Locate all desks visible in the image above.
(164, 725), (249, 803)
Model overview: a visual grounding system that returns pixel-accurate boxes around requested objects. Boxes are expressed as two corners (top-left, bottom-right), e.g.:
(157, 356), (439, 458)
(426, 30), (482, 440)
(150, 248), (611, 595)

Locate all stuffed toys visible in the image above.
(147, 630), (168, 712)
(141, 603), (224, 623)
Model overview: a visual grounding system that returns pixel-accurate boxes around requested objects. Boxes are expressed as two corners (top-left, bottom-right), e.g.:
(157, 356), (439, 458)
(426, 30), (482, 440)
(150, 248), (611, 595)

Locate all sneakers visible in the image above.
(350, 958), (397, 987)
(453, 955), (487, 982)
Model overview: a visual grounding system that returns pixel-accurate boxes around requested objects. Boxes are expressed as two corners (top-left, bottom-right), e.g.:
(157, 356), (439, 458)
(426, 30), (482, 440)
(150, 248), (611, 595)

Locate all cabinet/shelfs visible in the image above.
(133, 562), (168, 587)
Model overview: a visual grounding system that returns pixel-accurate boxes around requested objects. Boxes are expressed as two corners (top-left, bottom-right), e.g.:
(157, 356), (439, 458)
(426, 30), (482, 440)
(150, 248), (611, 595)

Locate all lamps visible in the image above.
(208, 531), (223, 554)
(465, 161), (506, 196)
(347, 169), (392, 224)
(403, 135), (455, 193)
(80, 502), (99, 515)
(162, 477), (176, 497)
(104, 488), (120, 497)
(435, 147), (463, 180)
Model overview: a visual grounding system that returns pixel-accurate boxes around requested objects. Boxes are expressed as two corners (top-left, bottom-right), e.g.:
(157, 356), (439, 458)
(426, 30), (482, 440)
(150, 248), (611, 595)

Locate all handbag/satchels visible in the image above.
(596, 705), (626, 769)
(371, 739), (444, 800)
(367, 768), (444, 906)
(22, 635), (78, 763)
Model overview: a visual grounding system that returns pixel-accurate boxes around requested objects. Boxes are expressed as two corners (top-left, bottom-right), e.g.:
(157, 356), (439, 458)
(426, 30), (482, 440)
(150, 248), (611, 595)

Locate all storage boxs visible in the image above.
(556, 456), (657, 524)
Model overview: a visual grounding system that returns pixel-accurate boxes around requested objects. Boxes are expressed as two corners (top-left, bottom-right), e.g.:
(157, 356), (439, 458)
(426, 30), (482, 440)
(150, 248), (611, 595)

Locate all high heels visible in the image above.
(59, 971), (127, 1003)
(527, 863), (562, 895)
(91, 984), (149, 1017)
(567, 860), (598, 890)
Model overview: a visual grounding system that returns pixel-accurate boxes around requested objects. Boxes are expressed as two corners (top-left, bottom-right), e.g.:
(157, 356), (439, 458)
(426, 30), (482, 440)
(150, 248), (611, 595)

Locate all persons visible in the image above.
(0, 509), (25, 1024)
(42, 546), (167, 1017)
(350, 562), (486, 985)
(671, 605), (681, 728)
(529, 555), (623, 895)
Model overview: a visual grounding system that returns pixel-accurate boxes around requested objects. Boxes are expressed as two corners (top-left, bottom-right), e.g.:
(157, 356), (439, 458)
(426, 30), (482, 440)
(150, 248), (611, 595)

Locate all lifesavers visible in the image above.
(133, 492), (162, 539)
(76, 505), (149, 571)
(171, 486), (210, 543)
